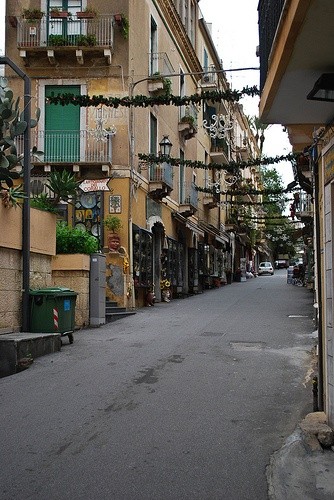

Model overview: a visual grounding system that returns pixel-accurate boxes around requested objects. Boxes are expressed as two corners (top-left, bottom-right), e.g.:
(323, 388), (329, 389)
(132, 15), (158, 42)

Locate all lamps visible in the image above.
(158, 134), (173, 158)
(306, 72), (334, 102)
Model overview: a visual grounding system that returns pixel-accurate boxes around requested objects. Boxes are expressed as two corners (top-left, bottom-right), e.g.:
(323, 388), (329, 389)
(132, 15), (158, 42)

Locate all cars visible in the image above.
(258, 262), (274, 275)
(277, 259), (289, 270)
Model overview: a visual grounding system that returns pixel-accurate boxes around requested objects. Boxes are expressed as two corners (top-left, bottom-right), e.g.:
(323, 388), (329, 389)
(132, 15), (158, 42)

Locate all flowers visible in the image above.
(159, 278), (171, 290)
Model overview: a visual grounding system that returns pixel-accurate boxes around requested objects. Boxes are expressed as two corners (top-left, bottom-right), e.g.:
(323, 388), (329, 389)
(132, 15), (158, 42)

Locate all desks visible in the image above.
(210, 276), (224, 288)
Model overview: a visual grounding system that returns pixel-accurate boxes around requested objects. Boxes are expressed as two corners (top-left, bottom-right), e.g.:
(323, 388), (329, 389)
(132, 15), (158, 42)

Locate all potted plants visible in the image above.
(103, 127), (116, 139)
(21, 6), (45, 25)
(50, 5), (73, 19)
(113, 12), (131, 40)
(47, 33), (97, 47)
(99, 214), (124, 252)
(76, 5), (98, 19)
(181, 114), (194, 124)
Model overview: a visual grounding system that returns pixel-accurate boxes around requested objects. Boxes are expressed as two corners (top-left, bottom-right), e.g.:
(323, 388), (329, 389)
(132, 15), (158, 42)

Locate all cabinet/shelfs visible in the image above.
(132, 229), (154, 288)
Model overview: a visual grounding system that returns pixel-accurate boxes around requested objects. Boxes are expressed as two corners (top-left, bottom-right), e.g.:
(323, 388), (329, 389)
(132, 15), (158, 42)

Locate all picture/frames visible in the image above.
(109, 194), (122, 214)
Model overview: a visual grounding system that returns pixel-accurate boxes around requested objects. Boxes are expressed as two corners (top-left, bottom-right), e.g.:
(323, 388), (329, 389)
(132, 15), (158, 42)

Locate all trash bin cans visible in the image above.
(21, 286), (80, 346)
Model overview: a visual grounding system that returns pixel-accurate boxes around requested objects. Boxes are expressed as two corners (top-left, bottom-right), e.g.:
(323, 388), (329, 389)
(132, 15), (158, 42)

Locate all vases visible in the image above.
(162, 288), (171, 303)
(145, 290), (154, 307)
(8, 15), (18, 28)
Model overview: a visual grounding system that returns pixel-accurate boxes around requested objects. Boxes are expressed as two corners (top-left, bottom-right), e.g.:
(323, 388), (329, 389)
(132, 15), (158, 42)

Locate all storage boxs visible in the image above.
(287, 278), (292, 284)
(306, 282), (314, 289)
(287, 268), (294, 274)
(288, 274), (293, 279)
(241, 277), (246, 281)
